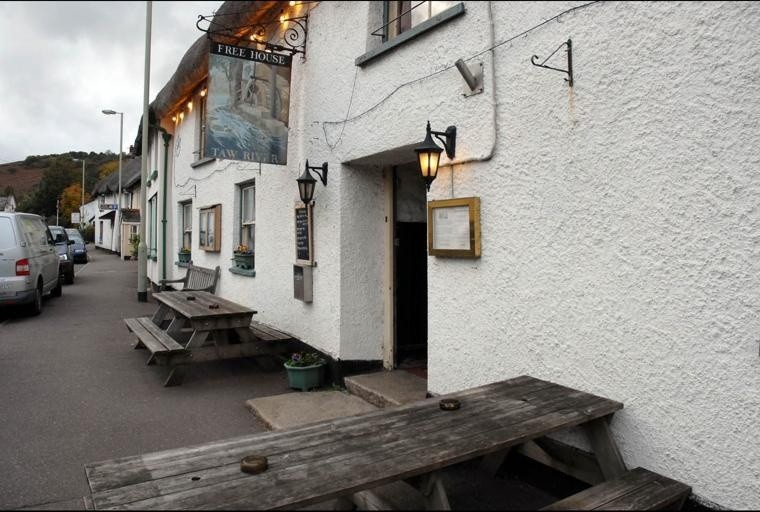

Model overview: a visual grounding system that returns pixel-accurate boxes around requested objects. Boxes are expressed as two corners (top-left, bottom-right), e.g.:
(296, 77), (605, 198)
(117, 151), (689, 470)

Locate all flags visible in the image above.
(283, 362), (322, 392)
(179, 253), (191, 262)
(230, 254), (254, 269)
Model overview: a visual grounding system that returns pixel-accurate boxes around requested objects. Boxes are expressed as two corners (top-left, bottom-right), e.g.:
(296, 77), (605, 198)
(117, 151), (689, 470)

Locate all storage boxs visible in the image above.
(158, 260), (220, 295)
(123, 317), (185, 366)
(535, 466), (692, 512)
(249, 321), (293, 342)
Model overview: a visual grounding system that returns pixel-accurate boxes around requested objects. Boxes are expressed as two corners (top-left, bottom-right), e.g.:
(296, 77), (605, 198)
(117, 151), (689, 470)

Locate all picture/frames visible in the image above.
(83, 375), (623, 511)
(151, 291), (257, 332)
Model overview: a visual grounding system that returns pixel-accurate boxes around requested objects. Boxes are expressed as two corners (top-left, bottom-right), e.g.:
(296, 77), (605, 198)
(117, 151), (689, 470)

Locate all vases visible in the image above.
(234, 245), (254, 254)
(286, 345), (326, 366)
(181, 248), (190, 253)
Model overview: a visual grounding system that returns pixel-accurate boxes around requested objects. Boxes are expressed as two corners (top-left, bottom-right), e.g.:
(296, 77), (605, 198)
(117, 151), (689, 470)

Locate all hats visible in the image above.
(46, 225), (90, 286)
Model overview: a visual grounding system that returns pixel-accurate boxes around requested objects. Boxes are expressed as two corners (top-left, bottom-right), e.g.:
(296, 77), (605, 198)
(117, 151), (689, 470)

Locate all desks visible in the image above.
(102, 107), (125, 251)
(71, 158), (85, 230)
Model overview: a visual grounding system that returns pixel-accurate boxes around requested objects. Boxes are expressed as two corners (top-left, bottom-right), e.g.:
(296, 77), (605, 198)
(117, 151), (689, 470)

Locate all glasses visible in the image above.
(413, 120), (456, 192)
(295, 159), (327, 215)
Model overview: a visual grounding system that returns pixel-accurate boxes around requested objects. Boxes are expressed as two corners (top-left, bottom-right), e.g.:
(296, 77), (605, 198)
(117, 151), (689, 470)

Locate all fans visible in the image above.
(294, 205), (313, 265)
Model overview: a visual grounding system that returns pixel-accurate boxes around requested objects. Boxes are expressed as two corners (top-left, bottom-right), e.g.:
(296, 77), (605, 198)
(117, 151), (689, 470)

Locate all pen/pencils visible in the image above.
(1, 210), (63, 317)
